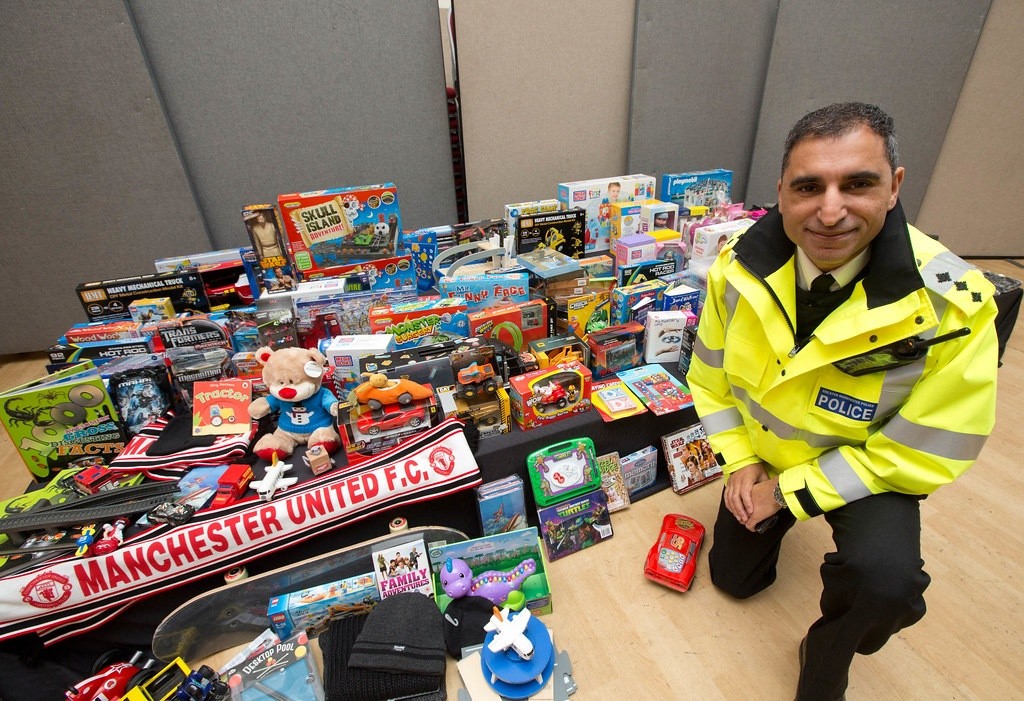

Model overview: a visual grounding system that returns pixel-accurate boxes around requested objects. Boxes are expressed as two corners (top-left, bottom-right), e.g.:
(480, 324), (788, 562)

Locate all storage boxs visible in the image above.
(429, 526), (553, 615)
(45, 170), (772, 496)
(266, 572), (381, 641)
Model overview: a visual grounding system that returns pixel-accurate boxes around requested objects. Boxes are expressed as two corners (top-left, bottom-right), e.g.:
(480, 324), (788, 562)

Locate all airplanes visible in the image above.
(248, 451), (299, 502)
(483, 604), (535, 661)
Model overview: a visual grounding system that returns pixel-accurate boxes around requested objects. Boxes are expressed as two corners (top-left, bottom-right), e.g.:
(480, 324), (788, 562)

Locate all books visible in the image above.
(0, 360), (119, 485)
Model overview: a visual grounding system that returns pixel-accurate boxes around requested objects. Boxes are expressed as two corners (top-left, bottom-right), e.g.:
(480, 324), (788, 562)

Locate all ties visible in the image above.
(806, 271), (837, 305)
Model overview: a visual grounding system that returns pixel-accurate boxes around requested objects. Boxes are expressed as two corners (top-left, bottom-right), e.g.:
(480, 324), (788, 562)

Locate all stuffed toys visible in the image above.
(248, 345), (344, 461)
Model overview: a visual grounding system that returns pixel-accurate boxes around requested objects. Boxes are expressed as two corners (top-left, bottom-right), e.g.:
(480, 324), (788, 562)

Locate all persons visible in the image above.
(684, 456), (706, 485)
(710, 234), (728, 257)
(269, 266), (295, 290)
(377, 547), (424, 581)
(602, 182), (623, 201)
(686, 103), (1001, 701)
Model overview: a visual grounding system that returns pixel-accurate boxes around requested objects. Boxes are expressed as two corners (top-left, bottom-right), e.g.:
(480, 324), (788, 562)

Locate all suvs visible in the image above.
(458, 362), (497, 398)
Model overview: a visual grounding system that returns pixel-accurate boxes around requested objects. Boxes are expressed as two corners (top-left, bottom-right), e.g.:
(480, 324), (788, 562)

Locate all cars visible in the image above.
(147, 501), (197, 528)
(64, 650), (155, 701)
(644, 513), (706, 592)
(534, 379), (567, 413)
(357, 400), (427, 437)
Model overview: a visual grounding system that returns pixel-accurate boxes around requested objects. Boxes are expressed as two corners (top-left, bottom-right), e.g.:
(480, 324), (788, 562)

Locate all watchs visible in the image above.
(772, 482), (790, 510)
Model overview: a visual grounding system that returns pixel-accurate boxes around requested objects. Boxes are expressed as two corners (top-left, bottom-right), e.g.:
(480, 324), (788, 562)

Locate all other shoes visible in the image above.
(794, 633), (846, 701)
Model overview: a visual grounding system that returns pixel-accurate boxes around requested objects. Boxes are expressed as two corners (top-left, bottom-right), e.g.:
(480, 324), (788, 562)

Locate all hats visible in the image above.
(348, 591), (446, 677)
(444, 592), (515, 660)
